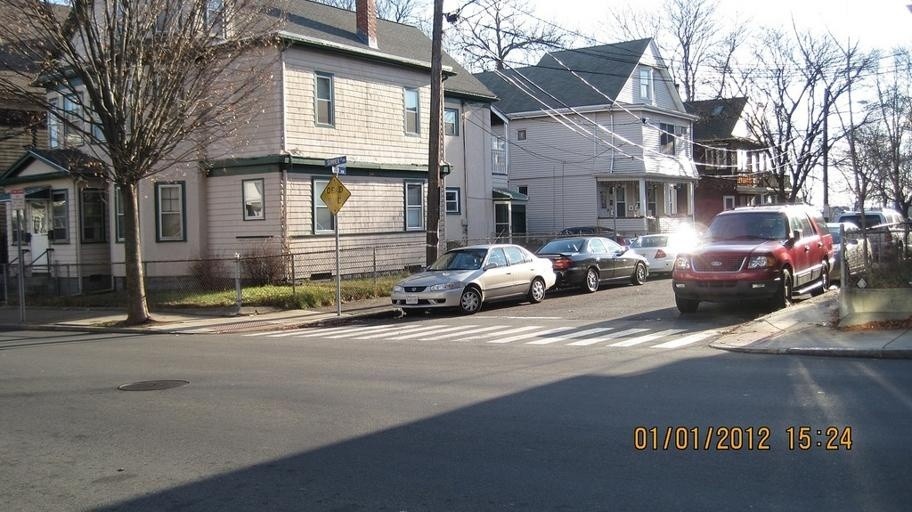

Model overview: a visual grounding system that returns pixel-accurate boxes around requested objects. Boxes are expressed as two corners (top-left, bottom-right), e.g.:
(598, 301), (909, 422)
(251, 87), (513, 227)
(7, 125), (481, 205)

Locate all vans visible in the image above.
(833, 206), (912, 262)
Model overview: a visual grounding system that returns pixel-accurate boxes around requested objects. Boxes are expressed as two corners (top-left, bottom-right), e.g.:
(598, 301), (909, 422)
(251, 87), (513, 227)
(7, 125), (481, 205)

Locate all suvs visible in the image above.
(672, 203), (834, 317)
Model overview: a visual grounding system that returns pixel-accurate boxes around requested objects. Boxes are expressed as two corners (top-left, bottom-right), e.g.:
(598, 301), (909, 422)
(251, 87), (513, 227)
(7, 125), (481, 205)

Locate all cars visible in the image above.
(825, 222), (876, 281)
(552, 227), (624, 244)
(390, 242), (556, 315)
(533, 233), (652, 295)
(627, 233), (697, 277)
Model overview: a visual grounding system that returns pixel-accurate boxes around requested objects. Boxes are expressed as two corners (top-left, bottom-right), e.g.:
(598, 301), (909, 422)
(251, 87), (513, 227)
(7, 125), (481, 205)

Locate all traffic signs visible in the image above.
(331, 163), (347, 176)
(323, 156), (350, 167)
